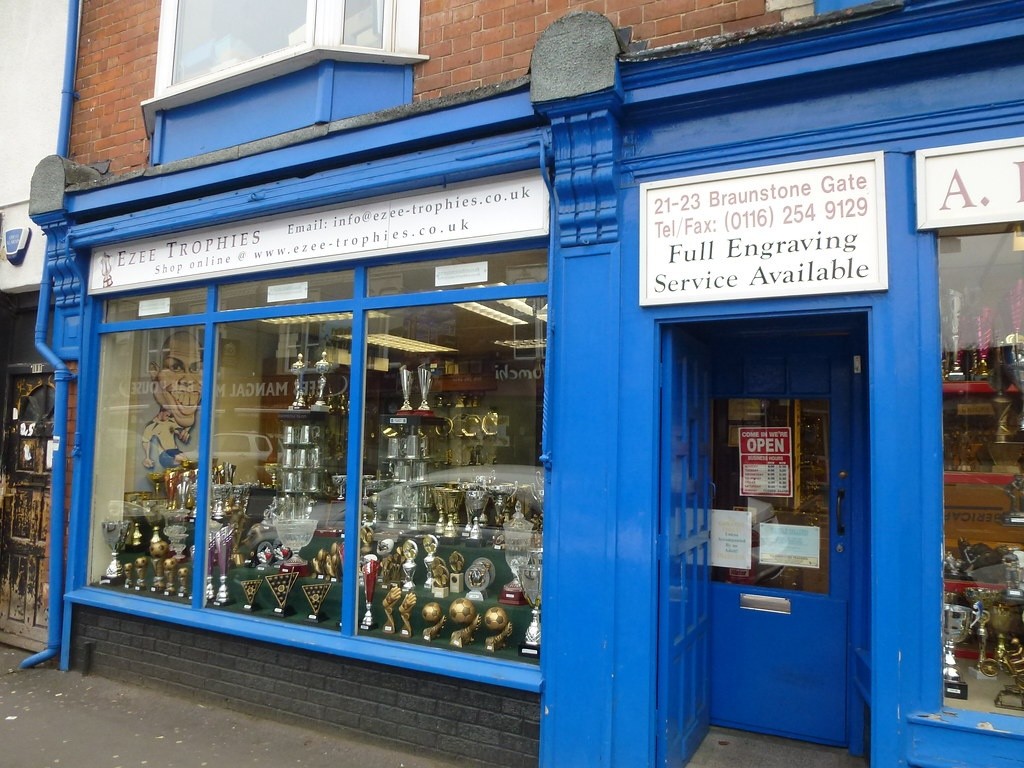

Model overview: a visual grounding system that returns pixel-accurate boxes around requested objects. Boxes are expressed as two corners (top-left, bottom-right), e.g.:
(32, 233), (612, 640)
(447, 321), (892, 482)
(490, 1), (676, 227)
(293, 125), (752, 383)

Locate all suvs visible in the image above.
(171, 431), (286, 486)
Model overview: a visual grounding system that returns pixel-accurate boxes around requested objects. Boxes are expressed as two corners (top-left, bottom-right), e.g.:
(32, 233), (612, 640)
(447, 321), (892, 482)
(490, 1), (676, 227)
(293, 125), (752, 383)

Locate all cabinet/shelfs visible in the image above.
(257, 374), (497, 486)
(942, 380), (1024, 665)
(376, 413), (452, 540)
(277, 410), (349, 500)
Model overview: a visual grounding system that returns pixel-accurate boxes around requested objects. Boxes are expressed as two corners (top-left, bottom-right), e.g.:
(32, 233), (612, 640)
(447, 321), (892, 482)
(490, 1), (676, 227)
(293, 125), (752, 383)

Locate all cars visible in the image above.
(296, 465), (545, 591)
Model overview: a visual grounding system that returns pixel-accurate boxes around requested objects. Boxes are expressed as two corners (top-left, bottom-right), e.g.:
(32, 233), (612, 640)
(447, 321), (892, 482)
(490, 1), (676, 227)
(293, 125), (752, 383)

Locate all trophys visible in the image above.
(100, 351), (543, 658)
(938, 278), (1024, 710)
